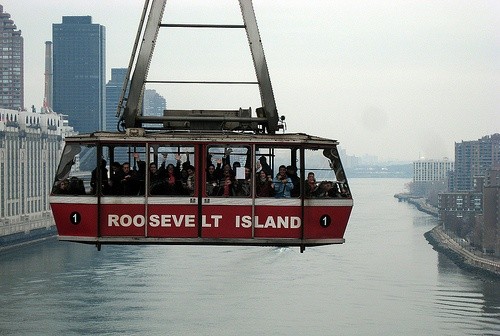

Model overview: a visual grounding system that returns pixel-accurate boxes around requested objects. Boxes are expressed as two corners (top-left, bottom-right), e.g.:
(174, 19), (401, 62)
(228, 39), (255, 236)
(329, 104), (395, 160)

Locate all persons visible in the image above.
(285, 166), (301, 198)
(271, 165), (294, 198)
(52, 176), (86, 196)
(109, 152), (195, 196)
(304, 172), (318, 198)
(206, 148), (277, 198)
(90, 158), (110, 195)
